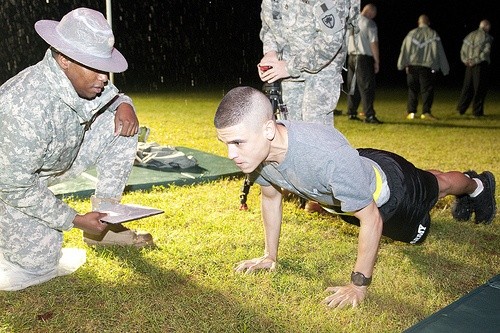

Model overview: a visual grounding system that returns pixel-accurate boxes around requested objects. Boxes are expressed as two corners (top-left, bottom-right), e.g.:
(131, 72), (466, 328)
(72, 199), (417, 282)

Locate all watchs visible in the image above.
(350, 271), (372, 286)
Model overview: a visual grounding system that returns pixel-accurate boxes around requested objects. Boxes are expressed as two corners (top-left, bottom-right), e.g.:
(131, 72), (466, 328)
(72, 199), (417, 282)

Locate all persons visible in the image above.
(348, 3), (384, 124)
(456, 21), (492, 117)
(214, 87), (496, 310)
(0, 8), (153, 279)
(397, 15), (449, 121)
(257, 0), (349, 214)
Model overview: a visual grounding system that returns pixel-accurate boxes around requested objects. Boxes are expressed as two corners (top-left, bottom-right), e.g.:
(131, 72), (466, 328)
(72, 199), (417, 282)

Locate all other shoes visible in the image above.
(349, 115), (363, 122)
(365, 116), (384, 124)
(406, 112), (415, 120)
(421, 113), (437, 120)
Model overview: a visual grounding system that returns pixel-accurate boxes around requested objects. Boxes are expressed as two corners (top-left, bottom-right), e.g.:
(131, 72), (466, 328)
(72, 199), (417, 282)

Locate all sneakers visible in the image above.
(471, 171), (496, 225)
(83, 223), (153, 247)
(452, 170), (477, 221)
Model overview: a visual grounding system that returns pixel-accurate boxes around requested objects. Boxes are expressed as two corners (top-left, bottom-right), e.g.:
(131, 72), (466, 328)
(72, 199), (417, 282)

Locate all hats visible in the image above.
(34, 8), (128, 73)
